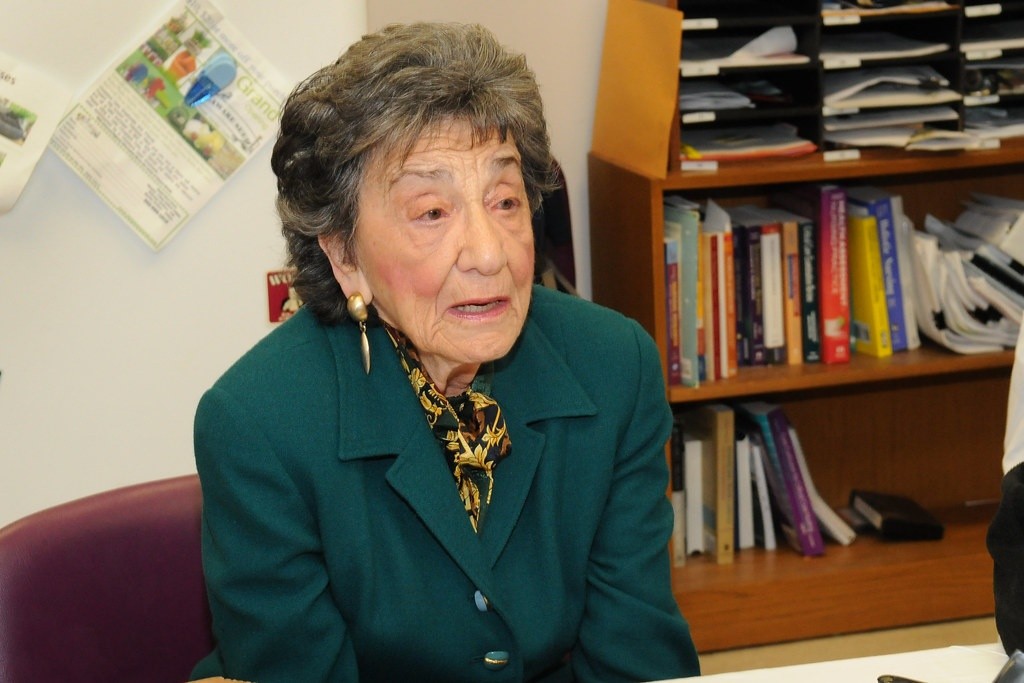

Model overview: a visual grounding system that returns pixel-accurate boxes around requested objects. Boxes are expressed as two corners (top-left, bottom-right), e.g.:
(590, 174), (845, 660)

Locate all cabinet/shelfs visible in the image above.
(636, 1), (824, 158)
(823, 1), (963, 149)
(588, 139), (1023, 654)
(962, 1), (1024, 131)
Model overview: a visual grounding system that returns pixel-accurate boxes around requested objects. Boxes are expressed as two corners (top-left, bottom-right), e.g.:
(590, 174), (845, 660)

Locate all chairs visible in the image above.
(0, 474), (216, 683)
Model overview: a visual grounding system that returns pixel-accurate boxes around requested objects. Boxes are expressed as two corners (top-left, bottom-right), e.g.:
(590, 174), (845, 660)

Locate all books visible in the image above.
(660, 193), (1024, 390)
(670, 399), (949, 566)
(676, 1), (1024, 161)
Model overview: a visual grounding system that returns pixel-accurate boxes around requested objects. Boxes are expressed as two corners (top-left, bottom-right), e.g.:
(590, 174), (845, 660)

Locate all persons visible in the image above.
(192, 23), (702, 682)
